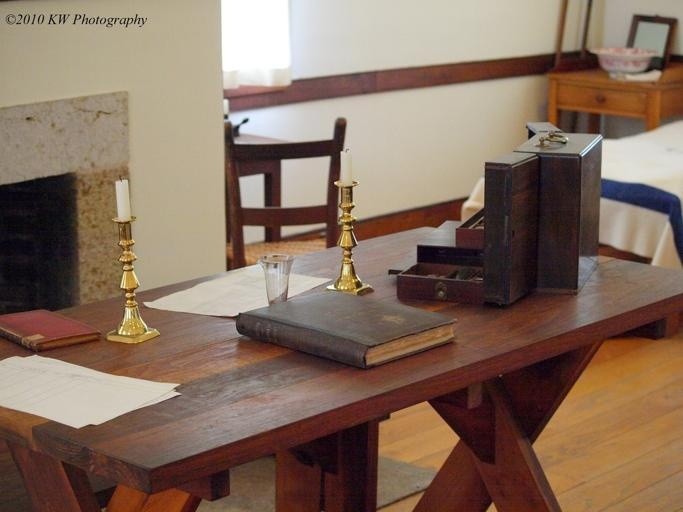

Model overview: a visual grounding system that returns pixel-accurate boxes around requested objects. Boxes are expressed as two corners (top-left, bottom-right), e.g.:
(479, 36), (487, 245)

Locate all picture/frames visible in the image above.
(625, 15), (677, 67)
(555, 0), (592, 72)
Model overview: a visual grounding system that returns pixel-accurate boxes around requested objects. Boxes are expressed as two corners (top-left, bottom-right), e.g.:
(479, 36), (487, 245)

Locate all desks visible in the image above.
(548, 63), (683, 132)
(226, 134), (281, 269)
(0, 227), (683, 512)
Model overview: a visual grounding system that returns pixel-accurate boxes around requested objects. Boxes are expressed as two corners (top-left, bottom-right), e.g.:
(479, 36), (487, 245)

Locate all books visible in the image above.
(0, 309), (100, 352)
(236, 287), (456, 368)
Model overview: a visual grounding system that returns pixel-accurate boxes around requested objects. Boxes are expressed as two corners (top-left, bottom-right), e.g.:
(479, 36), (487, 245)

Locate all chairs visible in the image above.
(225, 118), (346, 270)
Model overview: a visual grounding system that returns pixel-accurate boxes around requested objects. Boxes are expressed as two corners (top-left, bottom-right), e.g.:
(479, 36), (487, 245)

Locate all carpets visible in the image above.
(192, 454), (438, 512)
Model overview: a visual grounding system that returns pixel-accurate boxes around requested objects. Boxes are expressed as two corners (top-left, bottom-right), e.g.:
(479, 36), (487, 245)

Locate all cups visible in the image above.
(257, 254), (295, 306)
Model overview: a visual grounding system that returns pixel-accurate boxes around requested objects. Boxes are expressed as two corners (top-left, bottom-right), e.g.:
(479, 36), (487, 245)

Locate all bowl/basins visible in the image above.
(588, 45), (660, 74)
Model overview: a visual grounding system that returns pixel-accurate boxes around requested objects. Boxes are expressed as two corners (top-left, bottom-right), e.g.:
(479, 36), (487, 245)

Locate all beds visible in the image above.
(460, 121), (683, 338)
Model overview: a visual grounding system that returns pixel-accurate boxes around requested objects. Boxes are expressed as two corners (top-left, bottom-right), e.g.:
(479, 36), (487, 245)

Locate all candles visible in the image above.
(340, 147), (353, 185)
(115, 174), (131, 220)
